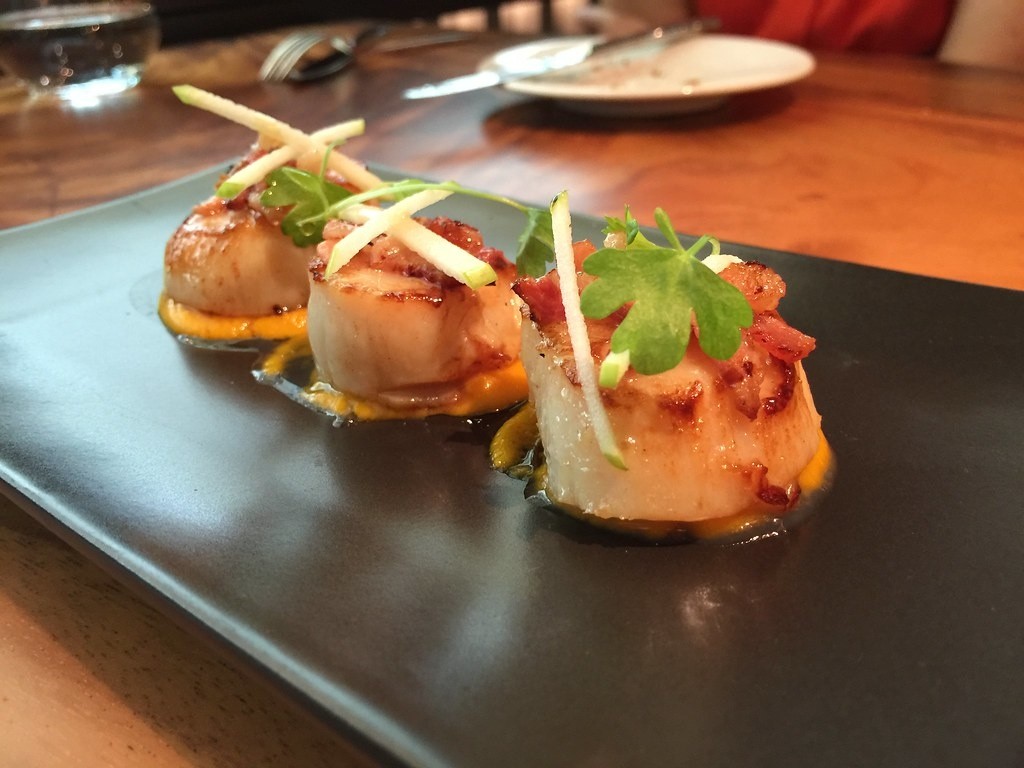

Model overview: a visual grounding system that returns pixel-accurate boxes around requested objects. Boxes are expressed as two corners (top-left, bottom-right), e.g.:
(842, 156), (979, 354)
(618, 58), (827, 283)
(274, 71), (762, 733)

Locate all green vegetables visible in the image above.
(256, 142), (755, 374)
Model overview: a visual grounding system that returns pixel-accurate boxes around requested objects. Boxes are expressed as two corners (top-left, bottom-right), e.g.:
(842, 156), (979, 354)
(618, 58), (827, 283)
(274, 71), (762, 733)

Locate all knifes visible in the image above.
(403, 16), (723, 100)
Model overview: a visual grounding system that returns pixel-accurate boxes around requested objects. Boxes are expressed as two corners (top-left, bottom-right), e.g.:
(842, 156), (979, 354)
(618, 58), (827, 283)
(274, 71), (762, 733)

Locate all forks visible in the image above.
(258, 28), (474, 83)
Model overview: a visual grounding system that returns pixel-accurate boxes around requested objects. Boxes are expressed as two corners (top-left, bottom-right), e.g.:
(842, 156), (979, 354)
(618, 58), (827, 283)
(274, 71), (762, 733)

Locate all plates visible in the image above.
(477, 36), (815, 120)
(1, 158), (1023, 768)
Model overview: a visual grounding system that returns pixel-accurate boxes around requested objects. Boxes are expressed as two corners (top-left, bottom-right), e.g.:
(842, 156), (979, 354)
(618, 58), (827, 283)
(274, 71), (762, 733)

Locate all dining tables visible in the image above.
(1, 1), (1024, 768)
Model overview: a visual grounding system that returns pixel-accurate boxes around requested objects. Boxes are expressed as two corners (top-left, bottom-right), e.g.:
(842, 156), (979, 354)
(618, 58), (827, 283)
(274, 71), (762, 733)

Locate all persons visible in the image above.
(581, 0), (958, 57)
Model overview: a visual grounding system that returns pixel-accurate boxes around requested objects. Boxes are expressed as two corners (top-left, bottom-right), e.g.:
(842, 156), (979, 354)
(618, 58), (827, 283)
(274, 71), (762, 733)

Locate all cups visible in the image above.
(0, 0), (161, 97)
